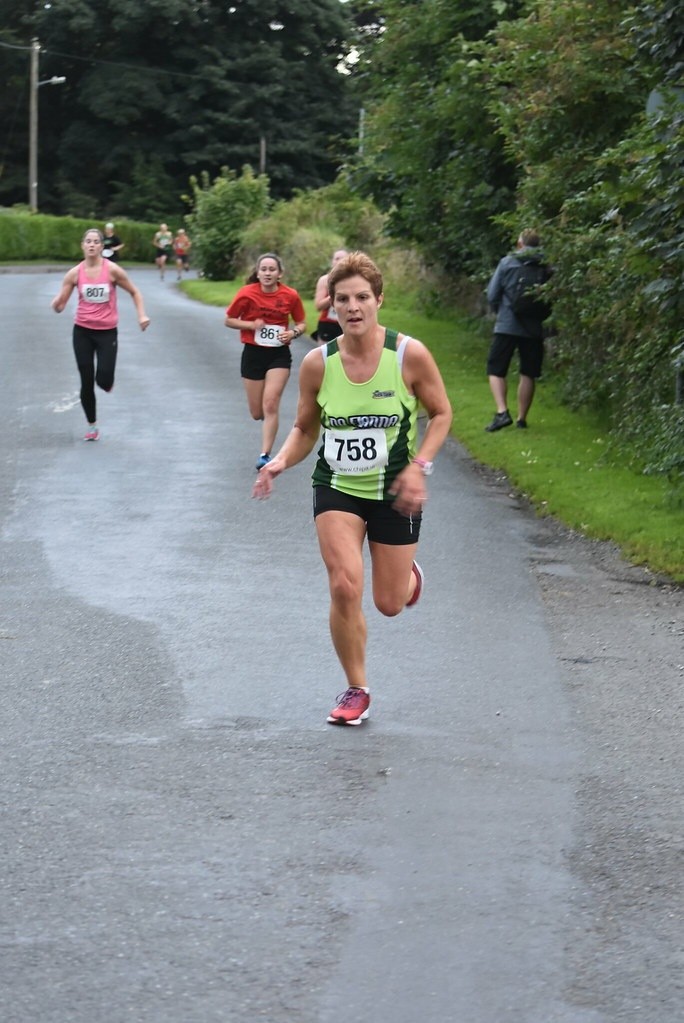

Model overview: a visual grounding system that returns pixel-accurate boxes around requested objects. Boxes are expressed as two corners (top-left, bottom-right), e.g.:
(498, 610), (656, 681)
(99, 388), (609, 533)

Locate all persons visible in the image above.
(310, 250), (348, 347)
(153, 223), (173, 282)
(172, 228), (192, 280)
(100, 223), (125, 263)
(250, 253), (453, 728)
(224, 252), (308, 471)
(483, 228), (559, 432)
(50, 228), (151, 443)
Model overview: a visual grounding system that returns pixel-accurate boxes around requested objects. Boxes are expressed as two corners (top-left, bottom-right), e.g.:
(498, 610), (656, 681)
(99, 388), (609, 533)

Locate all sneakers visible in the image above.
(403, 559), (423, 609)
(486, 410), (513, 432)
(255, 455), (272, 469)
(84, 426), (99, 441)
(518, 421), (528, 430)
(326, 687), (371, 727)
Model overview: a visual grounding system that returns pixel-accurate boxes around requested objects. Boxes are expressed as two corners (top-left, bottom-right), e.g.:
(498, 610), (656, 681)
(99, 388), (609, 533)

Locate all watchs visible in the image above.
(410, 458), (436, 478)
(291, 327), (300, 339)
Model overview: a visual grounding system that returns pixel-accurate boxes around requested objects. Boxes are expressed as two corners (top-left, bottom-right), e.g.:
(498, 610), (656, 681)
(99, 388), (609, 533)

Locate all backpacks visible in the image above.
(502, 253), (556, 325)
